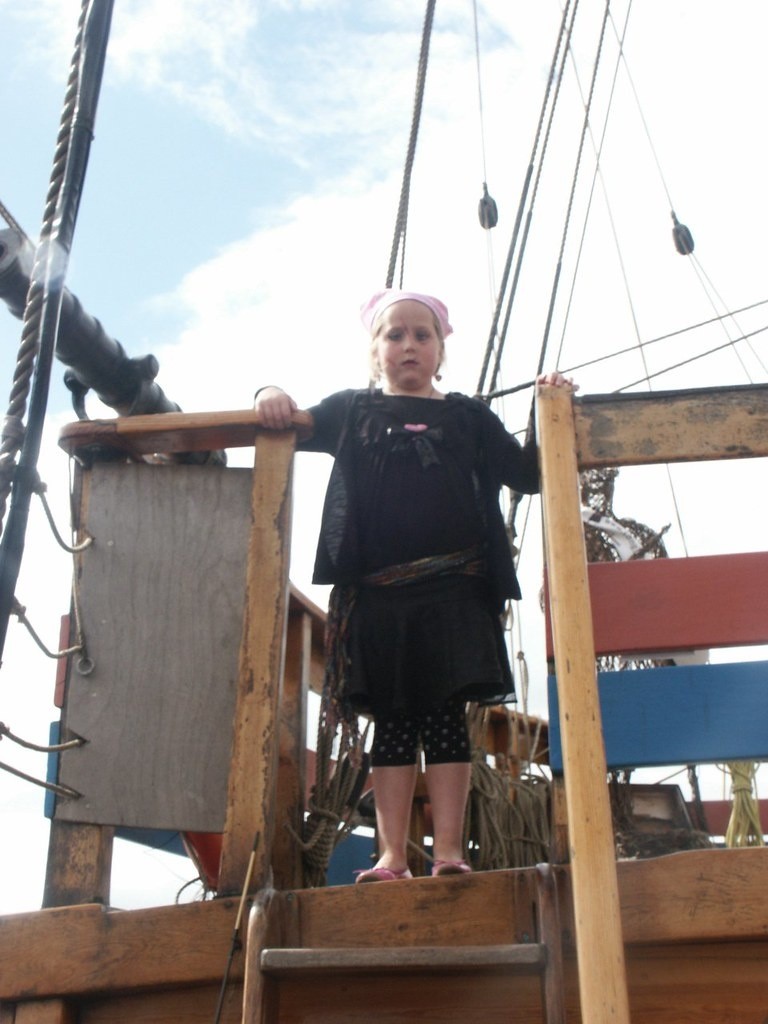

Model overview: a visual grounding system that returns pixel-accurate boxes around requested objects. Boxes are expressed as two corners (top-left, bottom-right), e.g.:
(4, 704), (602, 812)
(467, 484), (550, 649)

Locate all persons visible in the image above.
(255, 286), (582, 890)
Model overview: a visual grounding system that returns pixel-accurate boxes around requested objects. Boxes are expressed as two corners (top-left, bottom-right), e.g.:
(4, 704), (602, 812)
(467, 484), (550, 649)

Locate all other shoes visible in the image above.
(432, 859), (471, 876)
(357, 867), (414, 883)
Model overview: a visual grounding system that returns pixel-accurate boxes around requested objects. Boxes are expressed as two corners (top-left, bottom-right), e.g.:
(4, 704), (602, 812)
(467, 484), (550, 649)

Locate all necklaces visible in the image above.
(385, 386), (437, 432)
